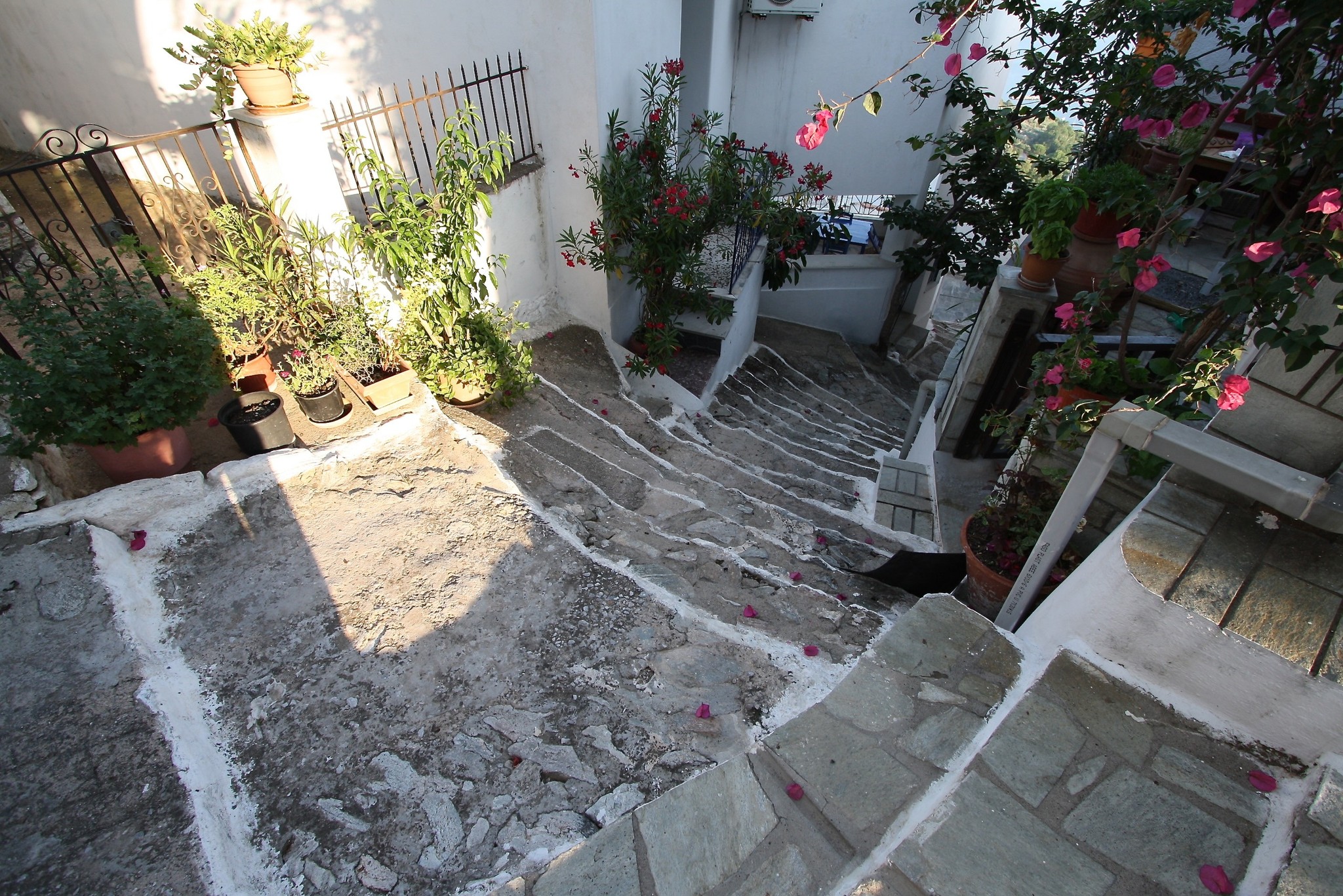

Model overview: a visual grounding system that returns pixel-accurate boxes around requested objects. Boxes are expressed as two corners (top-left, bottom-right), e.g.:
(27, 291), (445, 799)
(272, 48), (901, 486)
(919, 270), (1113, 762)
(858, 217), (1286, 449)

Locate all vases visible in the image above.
(217, 391), (297, 458)
(629, 329), (652, 358)
(960, 510), (1078, 632)
(294, 374), (345, 422)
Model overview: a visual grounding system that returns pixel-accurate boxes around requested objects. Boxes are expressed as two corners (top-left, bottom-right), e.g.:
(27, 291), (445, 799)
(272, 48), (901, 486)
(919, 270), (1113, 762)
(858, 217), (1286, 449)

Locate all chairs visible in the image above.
(822, 231), (853, 255)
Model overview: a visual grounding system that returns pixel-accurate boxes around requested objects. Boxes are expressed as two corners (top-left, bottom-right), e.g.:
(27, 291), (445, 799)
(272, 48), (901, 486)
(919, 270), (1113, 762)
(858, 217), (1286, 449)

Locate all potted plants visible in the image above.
(161, 2), (329, 160)
(1141, 102), (1213, 181)
(1, 99), (538, 488)
(1050, 354), (1150, 424)
(1070, 161), (1151, 245)
(1015, 177), (1088, 291)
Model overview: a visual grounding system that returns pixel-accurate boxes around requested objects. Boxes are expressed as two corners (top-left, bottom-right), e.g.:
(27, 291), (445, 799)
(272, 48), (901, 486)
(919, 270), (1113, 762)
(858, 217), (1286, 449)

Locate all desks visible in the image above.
(796, 211), (873, 254)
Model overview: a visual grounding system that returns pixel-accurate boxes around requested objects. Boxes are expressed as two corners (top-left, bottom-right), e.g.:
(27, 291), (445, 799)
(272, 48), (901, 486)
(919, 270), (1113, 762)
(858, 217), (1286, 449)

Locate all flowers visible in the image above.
(975, 143), (1300, 586)
(555, 57), (769, 380)
(274, 346), (334, 399)
